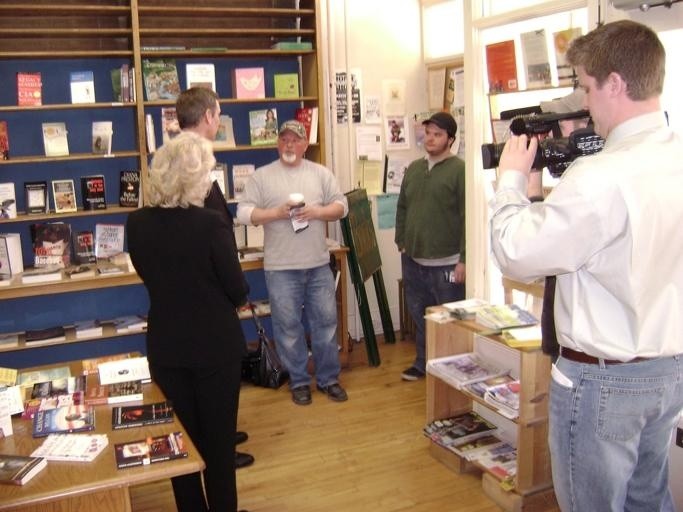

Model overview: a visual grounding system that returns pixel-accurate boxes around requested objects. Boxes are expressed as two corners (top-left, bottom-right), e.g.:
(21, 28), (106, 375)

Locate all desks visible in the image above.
(0, 351), (206, 512)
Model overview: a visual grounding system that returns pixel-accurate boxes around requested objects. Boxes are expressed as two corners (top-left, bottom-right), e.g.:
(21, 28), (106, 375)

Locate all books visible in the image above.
(144, 106), (319, 151)
(0, 121), (112, 160)
(0, 354), (189, 486)
(142, 58), (299, 101)
(1, 170), (142, 219)
(484, 25), (582, 144)
(239, 299), (272, 318)
(0, 222), (135, 286)
(421, 296), (542, 493)
(211, 163), (264, 263)
(18, 64), (137, 106)
(1, 315), (148, 348)
(141, 41), (312, 52)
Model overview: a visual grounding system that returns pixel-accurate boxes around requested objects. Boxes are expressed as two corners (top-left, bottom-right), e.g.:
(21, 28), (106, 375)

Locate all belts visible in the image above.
(559, 346), (660, 365)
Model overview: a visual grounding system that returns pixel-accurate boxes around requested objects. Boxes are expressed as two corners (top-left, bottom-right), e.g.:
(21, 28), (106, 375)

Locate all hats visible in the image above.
(421, 111), (457, 137)
(278, 119), (307, 139)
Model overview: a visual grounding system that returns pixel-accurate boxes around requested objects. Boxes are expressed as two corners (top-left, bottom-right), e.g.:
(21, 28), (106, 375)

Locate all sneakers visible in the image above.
(315, 382), (348, 404)
(401, 365), (426, 383)
(235, 450), (256, 469)
(236, 431), (248, 444)
(291, 384), (313, 407)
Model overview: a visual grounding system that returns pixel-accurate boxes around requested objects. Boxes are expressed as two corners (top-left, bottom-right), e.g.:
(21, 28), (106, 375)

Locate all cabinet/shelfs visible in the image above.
(425, 302), (558, 512)
(0, 0), (351, 367)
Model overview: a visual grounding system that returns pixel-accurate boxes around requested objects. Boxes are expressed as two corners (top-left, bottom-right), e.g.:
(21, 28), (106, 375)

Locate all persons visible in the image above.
(527, 117), (591, 365)
(394, 111), (465, 381)
(235, 119), (351, 406)
(390, 120), (401, 142)
(125, 130), (250, 512)
(488, 18), (683, 511)
